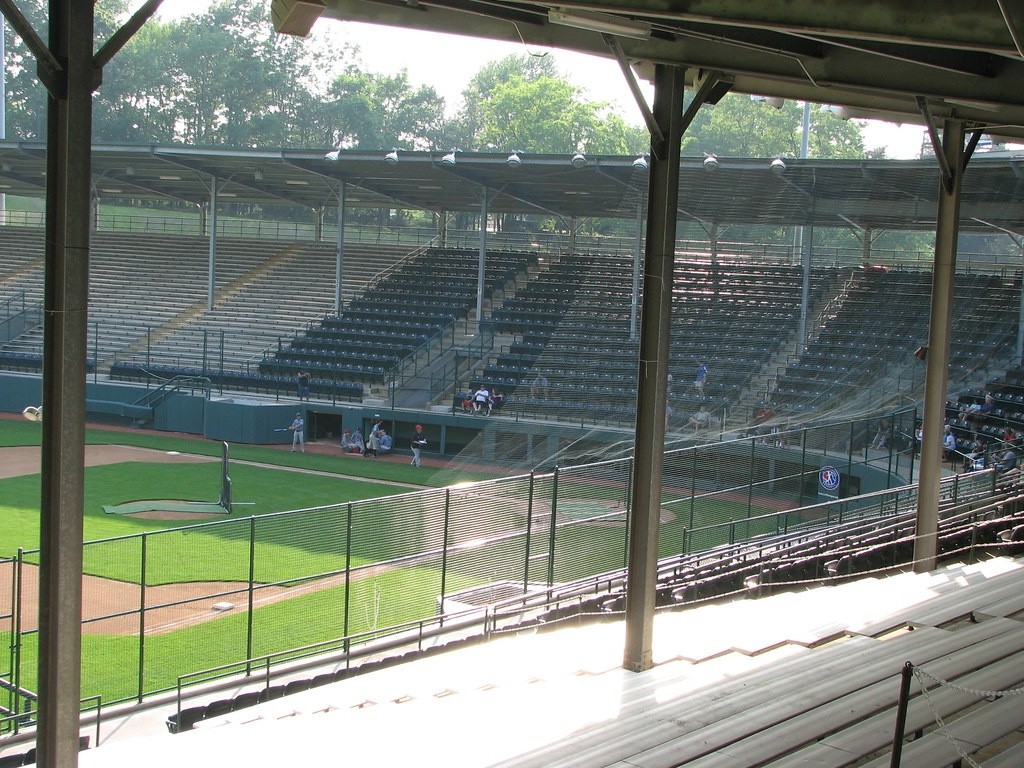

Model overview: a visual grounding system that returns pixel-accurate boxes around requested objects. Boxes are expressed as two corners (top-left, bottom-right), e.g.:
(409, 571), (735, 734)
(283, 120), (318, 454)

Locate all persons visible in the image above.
(410, 424), (427, 468)
(461, 389), (475, 415)
(756, 401), (787, 449)
(689, 406), (712, 434)
(530, 373), (549, 400)
(289, 412), (305, 453)
(298, 368), (310, 402)
(665, 400), (673, 430)
(341, 420), (393, 457)
(472, 384), (489, 413)
(485, 388), (503, 417)
(667, 373), (673, 394)
(869, 393), (1018, 478)
(694, 358), (709, 400)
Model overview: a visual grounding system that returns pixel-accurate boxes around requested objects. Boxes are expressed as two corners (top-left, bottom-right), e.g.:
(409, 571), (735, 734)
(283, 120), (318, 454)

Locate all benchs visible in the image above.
(0, 225), (1024, 768)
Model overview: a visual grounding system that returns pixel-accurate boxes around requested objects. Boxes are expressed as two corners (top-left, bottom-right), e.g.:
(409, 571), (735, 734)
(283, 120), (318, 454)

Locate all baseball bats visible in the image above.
(274, 429), (288, 432)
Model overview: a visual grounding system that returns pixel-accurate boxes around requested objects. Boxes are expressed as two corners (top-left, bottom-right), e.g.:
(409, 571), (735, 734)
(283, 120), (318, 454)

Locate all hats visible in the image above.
(356, 427), (360, 429)
(416, 425), (423, 429)
(296, 413), (300, 416)
